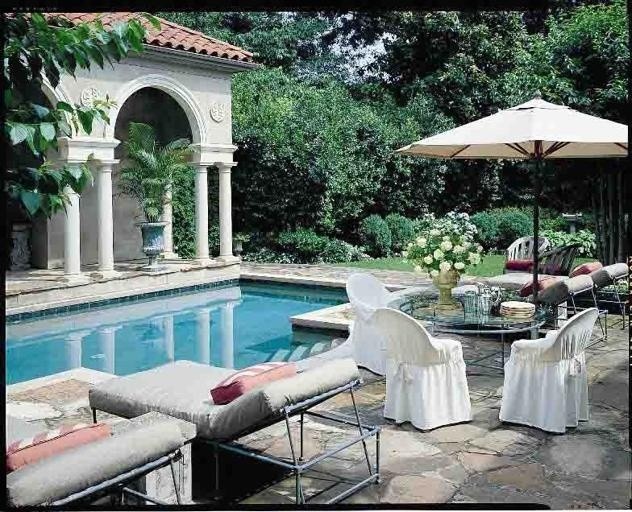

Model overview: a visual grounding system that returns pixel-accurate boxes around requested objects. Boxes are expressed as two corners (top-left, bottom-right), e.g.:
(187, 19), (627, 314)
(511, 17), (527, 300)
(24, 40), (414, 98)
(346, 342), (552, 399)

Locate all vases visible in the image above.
(432, 273), (459, 305)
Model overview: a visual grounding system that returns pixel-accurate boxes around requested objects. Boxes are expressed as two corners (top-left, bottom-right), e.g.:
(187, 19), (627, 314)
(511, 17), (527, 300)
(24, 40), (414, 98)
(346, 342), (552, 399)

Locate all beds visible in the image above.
(486, 236), (629, 433)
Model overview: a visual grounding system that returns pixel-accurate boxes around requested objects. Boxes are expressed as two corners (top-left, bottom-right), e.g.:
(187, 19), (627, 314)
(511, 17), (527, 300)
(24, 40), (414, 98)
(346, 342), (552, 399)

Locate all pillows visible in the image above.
(209, 361), (297, 404)
(6, 422), (112, 472)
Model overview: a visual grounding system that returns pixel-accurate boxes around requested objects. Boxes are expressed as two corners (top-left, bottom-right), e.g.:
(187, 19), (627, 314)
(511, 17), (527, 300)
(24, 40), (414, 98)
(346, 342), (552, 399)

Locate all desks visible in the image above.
(389, 294), (564, 366)
(112, 411), (198, 504)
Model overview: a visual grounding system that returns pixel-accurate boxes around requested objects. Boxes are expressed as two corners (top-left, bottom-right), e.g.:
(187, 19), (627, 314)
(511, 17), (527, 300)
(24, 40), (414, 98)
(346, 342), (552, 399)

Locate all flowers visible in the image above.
(398, 211), (491, 279)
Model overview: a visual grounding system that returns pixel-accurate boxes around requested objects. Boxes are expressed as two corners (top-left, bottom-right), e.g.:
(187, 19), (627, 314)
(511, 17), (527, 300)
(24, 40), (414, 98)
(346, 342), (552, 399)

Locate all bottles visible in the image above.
(464, 285), (493, 315)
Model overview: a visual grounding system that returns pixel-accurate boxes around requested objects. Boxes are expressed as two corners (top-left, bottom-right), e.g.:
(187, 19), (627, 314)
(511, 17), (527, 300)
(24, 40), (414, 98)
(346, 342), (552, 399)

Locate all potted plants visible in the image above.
(117, 121), (200, 273)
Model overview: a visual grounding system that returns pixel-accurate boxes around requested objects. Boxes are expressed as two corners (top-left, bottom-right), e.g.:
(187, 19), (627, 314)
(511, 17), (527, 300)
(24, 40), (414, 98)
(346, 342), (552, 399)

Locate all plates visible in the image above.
(499, 300), (536, 319)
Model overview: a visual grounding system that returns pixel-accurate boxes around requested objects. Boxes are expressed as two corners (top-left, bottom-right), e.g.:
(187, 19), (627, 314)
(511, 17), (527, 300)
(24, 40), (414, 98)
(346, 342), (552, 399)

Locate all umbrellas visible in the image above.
(390, 89), (629, 301)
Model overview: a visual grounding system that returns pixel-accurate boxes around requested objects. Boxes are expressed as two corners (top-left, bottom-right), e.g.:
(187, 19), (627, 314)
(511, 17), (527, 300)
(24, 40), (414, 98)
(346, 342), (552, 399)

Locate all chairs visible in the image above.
(374, 307), (474, 431)
(8, 421), (184, 505)
(88, 357), (382, 504)
(346, 272), (389, 375)
